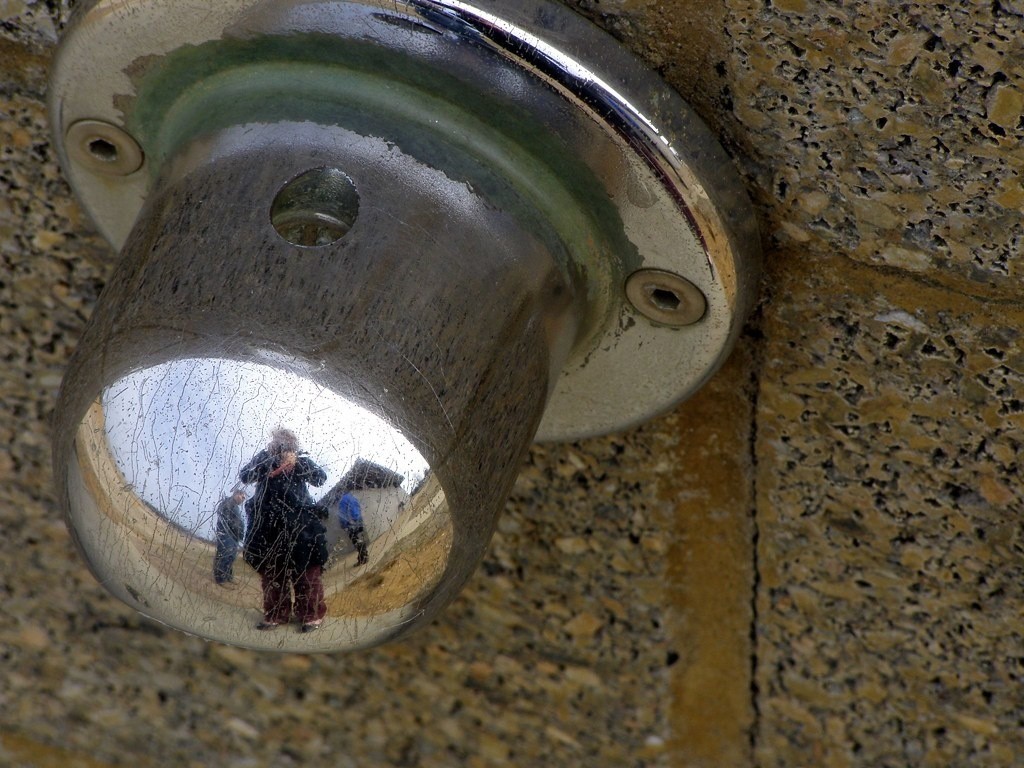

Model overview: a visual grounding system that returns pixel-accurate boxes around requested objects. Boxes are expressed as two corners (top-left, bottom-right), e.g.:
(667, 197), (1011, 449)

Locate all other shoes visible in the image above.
(258, 619), (288, 630)
(220, 579), (239, 590)
(302, 619), (324, 632)
(355, 562), (361, 567)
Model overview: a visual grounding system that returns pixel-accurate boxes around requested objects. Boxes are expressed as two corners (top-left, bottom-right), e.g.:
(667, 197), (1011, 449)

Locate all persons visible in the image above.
(337, 484), (371, 567)
(239, 428), (328, 633)
(212, 490), (246, 589)
